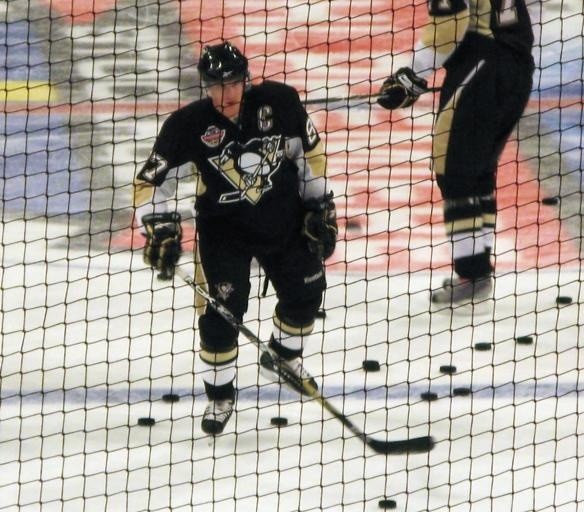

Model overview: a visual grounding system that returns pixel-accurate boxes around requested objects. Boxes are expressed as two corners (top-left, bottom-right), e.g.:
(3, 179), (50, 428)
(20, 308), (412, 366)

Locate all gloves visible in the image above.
(301, 192), (337, 260)
(376, 67), (428, 110)
(142, 212), (183, 281)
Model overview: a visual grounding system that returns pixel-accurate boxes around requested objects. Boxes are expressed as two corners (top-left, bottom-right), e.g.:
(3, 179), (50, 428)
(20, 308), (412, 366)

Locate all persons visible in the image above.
(130, 41), (337, 435)
(380, 1), (534, 305)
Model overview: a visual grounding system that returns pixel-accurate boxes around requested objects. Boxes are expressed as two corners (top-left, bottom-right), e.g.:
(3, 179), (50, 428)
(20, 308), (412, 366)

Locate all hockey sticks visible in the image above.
(175, 263), (436, 454)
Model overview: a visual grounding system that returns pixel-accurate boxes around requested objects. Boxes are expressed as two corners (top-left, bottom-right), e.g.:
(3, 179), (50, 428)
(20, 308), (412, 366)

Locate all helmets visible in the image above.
(197, 41), (248, 85)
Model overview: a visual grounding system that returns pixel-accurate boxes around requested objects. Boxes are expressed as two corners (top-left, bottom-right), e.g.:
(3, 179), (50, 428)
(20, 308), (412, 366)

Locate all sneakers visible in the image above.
(432, 275), (494, 304)
(201, 399), (237, 434)
(260, 347), (319, 397)
